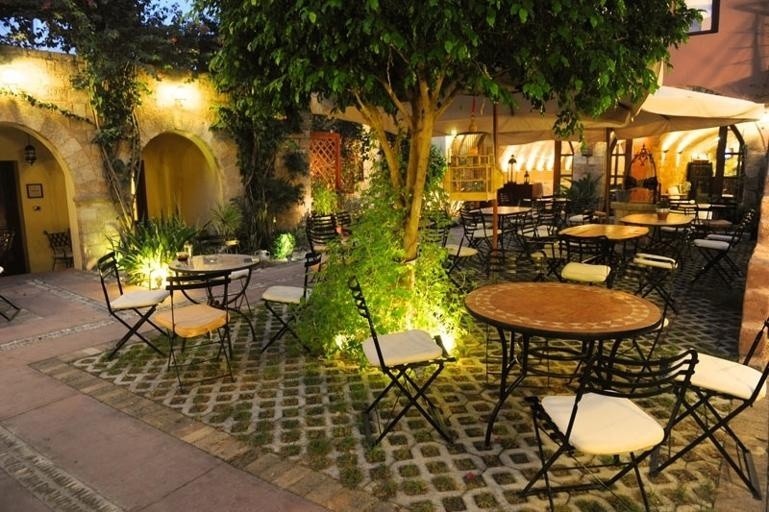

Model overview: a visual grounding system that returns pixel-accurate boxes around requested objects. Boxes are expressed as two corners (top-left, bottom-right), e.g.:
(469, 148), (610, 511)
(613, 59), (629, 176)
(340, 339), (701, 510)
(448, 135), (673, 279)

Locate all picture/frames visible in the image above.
(28, 183), (43, 198)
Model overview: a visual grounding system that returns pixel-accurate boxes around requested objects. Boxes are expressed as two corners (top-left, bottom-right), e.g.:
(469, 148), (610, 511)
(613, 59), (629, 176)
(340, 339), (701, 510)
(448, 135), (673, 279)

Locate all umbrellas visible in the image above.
(307, 47), (664, 263)
(484, 86), (765, 223)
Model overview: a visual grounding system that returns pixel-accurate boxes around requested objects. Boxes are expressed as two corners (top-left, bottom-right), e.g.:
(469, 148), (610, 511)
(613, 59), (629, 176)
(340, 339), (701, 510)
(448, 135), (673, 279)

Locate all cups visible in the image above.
(176, 241), (193, 267)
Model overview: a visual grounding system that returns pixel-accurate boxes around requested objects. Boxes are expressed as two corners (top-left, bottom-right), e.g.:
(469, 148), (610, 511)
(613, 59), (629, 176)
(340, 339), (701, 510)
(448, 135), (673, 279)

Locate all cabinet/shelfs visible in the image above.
(447, 154), (496, 202)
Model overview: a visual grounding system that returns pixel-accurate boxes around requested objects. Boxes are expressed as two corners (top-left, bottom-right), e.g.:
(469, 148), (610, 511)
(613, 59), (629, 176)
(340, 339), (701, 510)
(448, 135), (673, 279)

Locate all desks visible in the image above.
(167, 254), (262, 351)
(460, 278), (666, 465)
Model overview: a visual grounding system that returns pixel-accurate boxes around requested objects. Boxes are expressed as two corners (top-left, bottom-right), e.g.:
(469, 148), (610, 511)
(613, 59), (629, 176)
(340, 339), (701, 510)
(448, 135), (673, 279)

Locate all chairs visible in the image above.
(156, 273), (235, 391)
(349, 275), (458, 452)
(45, 228), (75, 272)
(0, 225), (19, 321)
(523, 346), (700, 511)
(260, 246), (325, 356)
(96, 250), (173, 359)
(649, 314), (769, 501)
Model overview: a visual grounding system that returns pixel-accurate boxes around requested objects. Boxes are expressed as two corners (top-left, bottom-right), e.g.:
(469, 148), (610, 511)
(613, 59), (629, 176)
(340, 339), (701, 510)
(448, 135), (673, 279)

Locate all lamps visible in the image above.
(24, 134), (36, 166)
